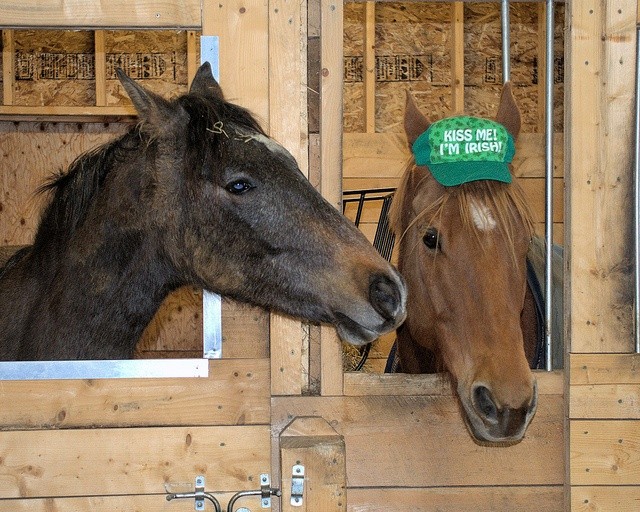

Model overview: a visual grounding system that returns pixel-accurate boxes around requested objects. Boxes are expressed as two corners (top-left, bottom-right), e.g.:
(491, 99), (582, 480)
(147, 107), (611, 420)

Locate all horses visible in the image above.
(0, 62), (408, 361)
(387, 80), (538, 448)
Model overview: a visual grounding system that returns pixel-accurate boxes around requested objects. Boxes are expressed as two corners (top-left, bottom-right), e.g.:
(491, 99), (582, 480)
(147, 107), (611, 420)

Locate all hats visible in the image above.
(412, 116), (516, 187)
